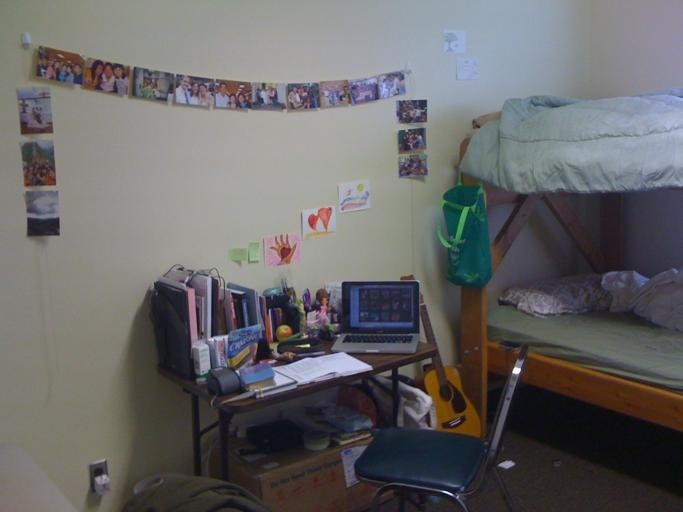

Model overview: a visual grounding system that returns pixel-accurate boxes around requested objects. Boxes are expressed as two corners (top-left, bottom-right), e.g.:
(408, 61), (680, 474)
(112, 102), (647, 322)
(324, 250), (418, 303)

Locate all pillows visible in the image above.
(498, 276), (611, 318)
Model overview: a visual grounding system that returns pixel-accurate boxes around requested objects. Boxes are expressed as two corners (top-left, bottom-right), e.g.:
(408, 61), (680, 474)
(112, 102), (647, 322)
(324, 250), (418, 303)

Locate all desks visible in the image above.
(158, 335), (438, 484)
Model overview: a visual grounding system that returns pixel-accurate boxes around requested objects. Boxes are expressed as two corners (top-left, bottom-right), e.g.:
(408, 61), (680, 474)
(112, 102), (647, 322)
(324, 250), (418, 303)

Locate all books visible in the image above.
(154, 266), (374, 399)
(309, 419), (373, 446)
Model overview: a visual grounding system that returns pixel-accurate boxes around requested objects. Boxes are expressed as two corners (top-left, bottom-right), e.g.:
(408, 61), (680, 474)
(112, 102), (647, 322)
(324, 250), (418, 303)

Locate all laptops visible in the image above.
(330, 280), (421, 354)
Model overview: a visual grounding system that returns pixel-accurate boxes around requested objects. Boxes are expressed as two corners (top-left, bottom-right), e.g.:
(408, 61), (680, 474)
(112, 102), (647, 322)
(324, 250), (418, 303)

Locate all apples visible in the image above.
(276, 325), (293, 342)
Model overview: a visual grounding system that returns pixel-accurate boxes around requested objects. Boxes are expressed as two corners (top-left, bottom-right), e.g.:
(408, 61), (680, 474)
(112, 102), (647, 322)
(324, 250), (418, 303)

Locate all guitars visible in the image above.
(400, 275), (481, 437)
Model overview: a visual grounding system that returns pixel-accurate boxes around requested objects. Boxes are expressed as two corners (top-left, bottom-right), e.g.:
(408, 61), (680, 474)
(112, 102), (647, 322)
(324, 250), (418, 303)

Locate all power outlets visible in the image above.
(89, 460), (106, 492)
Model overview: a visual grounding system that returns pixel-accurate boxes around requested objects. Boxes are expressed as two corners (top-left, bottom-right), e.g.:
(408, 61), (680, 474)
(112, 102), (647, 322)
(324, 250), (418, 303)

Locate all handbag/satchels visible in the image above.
(436, 171), (492, 288)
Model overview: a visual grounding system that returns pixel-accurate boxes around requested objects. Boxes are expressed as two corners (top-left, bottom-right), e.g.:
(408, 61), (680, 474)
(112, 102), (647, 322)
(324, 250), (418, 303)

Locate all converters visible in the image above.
(93, 474), (112, 495)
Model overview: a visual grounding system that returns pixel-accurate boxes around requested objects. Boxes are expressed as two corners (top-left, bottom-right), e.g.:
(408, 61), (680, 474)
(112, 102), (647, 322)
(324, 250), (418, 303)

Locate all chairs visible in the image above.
(355, 344), (528, 512)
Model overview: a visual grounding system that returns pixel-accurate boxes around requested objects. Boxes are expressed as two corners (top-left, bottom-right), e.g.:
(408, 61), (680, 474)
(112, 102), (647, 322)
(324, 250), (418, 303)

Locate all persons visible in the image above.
(406, 154), (422, 172)
(316, 295), (330, 326)
(37, 52), (406, 109)
(405, 131), (425, 152)
(19, 98), (50, 129)
(23, 161), (56, 186)
(396, 100), (428, 123)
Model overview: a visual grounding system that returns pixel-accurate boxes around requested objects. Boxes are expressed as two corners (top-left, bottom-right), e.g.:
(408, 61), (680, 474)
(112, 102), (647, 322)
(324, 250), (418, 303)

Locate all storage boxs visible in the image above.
(225, 429), (396, 512)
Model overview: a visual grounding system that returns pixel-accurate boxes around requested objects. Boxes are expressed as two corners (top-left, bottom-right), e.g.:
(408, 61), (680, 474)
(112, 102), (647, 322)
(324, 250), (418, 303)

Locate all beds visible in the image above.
(461, 119), (683, 440)
